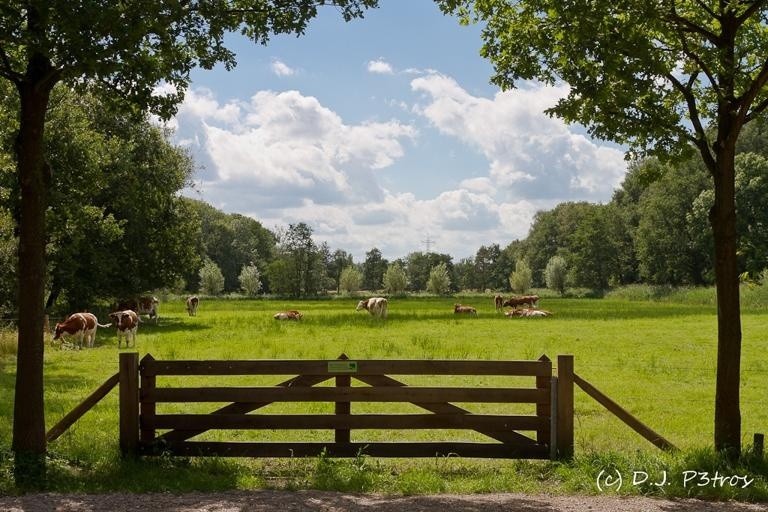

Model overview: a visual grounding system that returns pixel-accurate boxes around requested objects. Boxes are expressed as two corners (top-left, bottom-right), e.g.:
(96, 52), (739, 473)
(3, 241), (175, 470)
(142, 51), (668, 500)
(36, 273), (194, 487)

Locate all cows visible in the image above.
(356, 297), (388, 320)
(108, 309), (144, 349)
(494, 293), (554, 318)
(185, 296), (199, 317)
(53, 312), (112, 350)
(117, 296), (159, 326)
(453, 302), (477, 314)
(273, 310), (303, 322)
